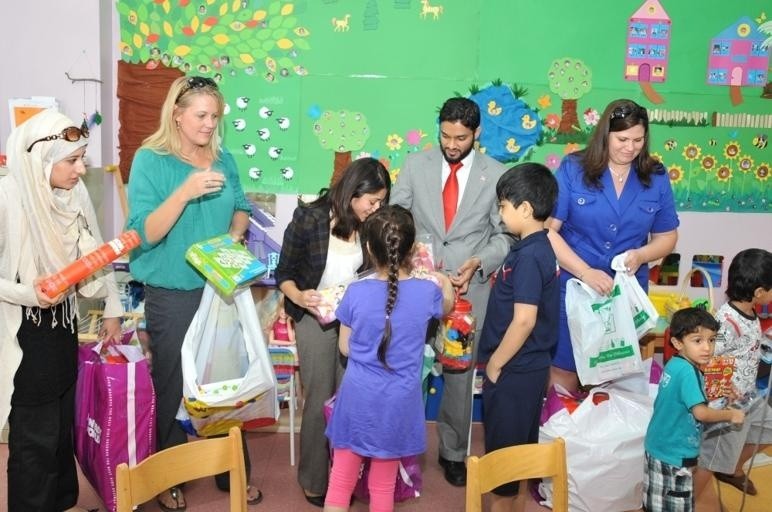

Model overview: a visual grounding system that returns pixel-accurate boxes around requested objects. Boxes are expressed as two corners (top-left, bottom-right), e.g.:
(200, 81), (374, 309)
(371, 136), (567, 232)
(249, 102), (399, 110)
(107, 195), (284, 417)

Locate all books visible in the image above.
(6, 95), (58, 133)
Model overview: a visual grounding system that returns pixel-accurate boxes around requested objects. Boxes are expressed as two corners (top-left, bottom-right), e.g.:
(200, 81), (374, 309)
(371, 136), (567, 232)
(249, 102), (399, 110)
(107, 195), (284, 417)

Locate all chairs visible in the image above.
(115, 427), (248, 512)
(467, 441), (568, 512)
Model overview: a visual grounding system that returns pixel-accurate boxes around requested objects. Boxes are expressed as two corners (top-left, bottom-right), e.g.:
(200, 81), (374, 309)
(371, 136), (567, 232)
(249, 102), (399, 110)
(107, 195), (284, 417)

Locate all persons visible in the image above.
(641, 304), (747, 512)
(473, 161), (560, 510)
(314, 205), (456, 512)
(276, 159), (392, 509)
(388, 94), (511, 487)
(537, 97), (679, 401)
(691, 247), (772, 501)
(265, 295), (299, 346)
(119, 73), (265, 512)
(0, 104), (128, 510)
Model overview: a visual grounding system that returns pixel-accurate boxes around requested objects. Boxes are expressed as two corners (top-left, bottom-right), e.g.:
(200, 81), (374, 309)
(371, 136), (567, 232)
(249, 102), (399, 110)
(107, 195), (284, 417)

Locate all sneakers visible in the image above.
(438, 456), (466, 486)
(714, 471), (757, 496)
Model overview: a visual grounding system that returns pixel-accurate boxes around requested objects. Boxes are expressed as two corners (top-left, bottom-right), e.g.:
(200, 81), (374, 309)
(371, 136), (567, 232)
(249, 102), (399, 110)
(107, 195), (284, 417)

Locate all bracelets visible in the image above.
(574, 267), (594, 277)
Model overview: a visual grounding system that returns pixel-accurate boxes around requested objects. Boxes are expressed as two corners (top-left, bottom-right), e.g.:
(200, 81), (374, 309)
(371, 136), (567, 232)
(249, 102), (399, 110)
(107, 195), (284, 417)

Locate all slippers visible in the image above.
(157, 487), (186, 512)
(247, 485), (263, 505)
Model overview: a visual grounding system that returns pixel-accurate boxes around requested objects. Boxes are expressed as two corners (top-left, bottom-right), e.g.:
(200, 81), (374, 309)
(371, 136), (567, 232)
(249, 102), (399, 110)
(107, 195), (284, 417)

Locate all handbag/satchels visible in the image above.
(324, 394), (422, 503)
(73, 334), (158, 512)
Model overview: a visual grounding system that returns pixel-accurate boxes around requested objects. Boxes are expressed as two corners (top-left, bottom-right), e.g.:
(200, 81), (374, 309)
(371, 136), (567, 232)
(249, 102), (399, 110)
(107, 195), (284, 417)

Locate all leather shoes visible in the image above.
(303, 487), (325, 508)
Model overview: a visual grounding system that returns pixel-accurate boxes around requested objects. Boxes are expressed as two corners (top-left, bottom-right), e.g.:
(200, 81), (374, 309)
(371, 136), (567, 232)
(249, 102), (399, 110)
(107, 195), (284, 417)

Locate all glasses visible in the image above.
(27, 122), (89, 152)
(610, 104), (647, 119)
(177, 77), (217, 104)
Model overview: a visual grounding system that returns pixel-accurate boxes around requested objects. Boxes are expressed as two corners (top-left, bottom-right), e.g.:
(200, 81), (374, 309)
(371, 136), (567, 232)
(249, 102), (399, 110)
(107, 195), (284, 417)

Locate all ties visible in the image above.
(443, 160), (464, 230)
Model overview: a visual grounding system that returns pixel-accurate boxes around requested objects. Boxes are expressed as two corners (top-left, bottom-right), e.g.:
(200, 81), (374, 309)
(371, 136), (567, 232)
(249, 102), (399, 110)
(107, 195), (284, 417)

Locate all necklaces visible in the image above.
(609, 168), (629, 183)
